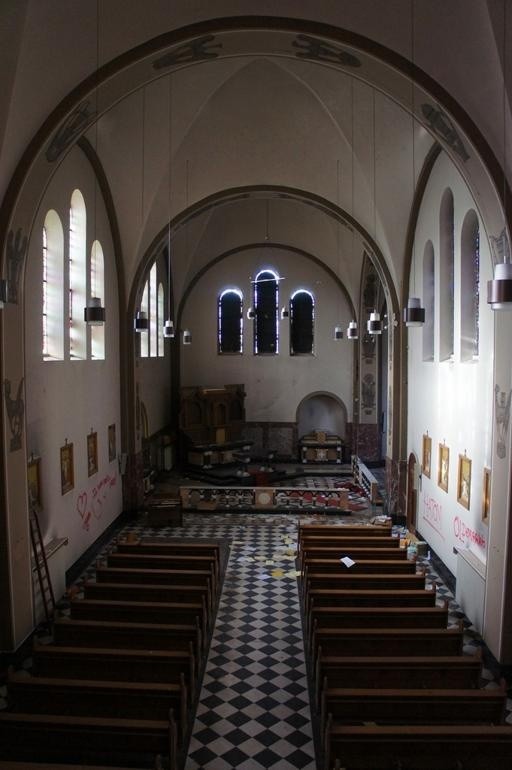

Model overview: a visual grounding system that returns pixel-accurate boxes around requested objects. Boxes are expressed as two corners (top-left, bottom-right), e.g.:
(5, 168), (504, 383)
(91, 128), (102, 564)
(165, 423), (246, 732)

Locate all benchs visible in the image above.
(296, 517), (511, 769)
(0, 536), (220, 770)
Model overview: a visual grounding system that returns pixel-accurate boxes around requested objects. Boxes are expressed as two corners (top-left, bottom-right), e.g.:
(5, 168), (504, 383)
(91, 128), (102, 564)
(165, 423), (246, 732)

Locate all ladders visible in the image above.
(28, 510), (57, 617)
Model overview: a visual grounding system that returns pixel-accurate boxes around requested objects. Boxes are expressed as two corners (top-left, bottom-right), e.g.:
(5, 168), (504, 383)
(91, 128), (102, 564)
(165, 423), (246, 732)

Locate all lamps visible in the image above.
(84, 1), (193, 346)
(487, 1), (511, 312)
(367, 91), (381, 335)
(334, 159), (343, 340)
(402, 2), (424, 325)
(347, 79), (358, 339)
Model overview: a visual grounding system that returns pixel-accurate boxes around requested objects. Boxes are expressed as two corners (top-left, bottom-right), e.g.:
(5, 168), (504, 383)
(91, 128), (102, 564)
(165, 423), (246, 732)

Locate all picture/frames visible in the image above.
(420, 434), (490, 528)
(27, 423), (117, 512)
